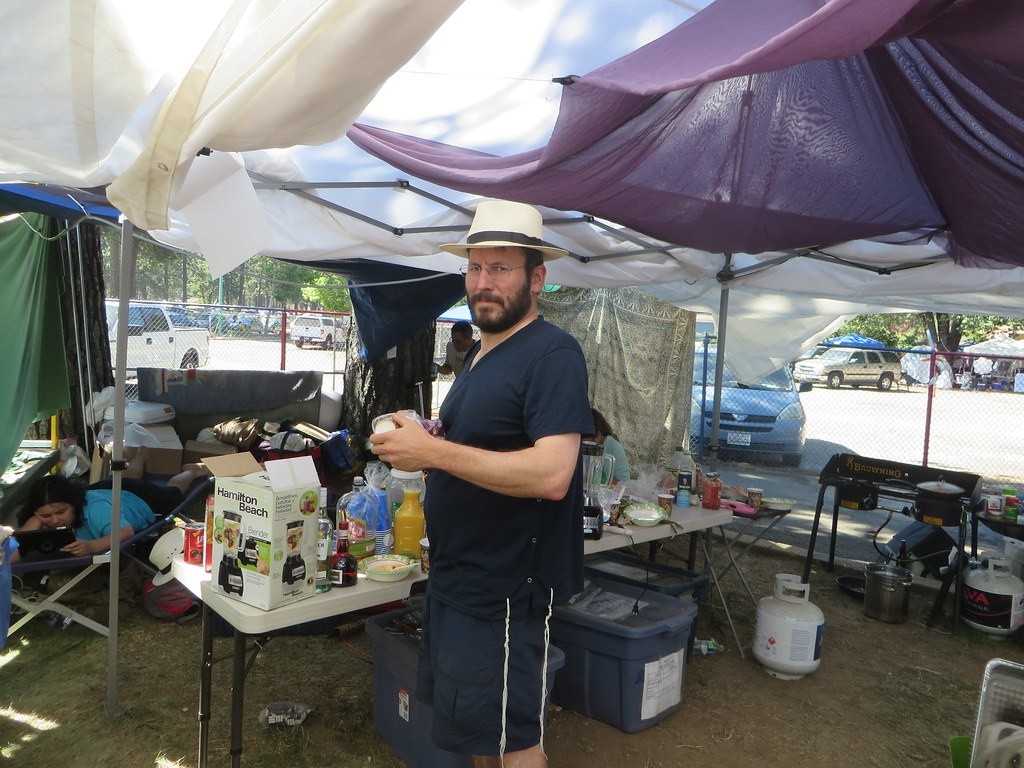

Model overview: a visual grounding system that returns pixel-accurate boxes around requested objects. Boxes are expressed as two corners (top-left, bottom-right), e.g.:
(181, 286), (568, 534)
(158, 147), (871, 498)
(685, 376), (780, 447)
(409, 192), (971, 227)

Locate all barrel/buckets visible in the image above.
(865, 562), (914, 625)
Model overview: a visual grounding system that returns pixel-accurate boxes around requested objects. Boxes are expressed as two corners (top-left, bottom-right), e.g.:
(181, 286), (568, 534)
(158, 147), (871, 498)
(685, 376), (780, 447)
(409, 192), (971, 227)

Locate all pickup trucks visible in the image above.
(105, 301), (210, 370)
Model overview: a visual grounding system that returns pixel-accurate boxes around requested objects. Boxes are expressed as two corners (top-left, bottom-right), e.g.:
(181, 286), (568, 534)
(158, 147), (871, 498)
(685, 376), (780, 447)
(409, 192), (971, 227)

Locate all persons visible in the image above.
(582, 408), (630, 489)
(6, 461), (210, 566)
(434, 320), (478, 380)
(369, 200), (596, 768)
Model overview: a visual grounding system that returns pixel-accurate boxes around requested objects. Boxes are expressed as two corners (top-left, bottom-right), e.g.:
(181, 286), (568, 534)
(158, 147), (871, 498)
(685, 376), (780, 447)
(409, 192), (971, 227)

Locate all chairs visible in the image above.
(968, 656), (1024, 767)
(2, 473), (215, 640)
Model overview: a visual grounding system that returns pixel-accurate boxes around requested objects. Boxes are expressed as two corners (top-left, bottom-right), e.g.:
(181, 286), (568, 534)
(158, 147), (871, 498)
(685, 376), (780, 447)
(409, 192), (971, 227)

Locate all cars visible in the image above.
(163, 305), (282, 334)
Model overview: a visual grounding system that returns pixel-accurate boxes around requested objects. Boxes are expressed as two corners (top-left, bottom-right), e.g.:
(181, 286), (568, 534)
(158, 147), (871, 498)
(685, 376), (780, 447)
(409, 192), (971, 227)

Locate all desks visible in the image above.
(169, 481), (734, 767)
(690, 491), (791, 657)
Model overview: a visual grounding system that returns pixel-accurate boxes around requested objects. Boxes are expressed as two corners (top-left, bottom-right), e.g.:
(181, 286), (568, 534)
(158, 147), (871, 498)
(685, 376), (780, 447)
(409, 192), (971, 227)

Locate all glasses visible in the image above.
(449, 337), (468, 342)
(459, 264), (525, 280)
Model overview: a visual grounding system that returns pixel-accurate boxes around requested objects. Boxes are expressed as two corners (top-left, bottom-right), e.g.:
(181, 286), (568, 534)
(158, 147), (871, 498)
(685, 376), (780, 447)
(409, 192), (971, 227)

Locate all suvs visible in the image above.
(793, 346), (903, 391)
(290, 315), (346, 351)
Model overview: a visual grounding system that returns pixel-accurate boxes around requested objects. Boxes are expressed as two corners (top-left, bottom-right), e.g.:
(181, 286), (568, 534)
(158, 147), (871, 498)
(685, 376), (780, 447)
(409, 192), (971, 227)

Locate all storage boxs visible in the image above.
(88, 423), (182, 486)
(991, 383), (1002, 390)
(976, 384), (986, 391)
(184, 439), (238, 472)
(548, 570), (701, 735)
(363, 602), (568, 767)
(199, 450), (323, 613)
(875, 519), (973, 585)
(583, 550), (711, 660)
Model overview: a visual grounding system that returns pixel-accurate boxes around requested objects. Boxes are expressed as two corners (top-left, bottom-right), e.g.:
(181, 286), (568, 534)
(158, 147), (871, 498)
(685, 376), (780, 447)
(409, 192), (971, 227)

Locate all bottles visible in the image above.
(987, 487), (1018, 521)
(678, 451), (696, 491)
(547, 701), (564, 723)
(895, 540), (908, 567)
(420, 538), (432, 574)
(669, 446), (684, 475)
(333, 522), (357, 588)
(335, 476), (377, 571)
(748, 488), (763, 511)
(658, 464), (723, 516)
(394, 488), (425, 558)
(692, 639), (724, 655)
(315, 487), (334, 594)
(384, 468), (427, 526)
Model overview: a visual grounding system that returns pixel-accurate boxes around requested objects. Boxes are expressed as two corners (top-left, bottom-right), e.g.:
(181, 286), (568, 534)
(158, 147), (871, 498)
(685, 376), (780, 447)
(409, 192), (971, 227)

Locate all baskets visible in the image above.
(368, 528), (392, 554)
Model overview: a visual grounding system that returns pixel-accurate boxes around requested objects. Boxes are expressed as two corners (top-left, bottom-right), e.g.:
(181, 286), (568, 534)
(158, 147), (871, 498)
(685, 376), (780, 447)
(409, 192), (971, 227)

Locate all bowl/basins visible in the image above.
(624, 501), (667, 526)
(359, 554), (415, 581)
(762, 497), (797, 511)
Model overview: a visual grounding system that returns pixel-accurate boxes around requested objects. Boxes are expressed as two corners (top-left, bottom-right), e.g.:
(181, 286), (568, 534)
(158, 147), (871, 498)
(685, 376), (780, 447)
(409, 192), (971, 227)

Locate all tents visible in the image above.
(0, 0), (1024, 708)
(963, 335), (1024, 391)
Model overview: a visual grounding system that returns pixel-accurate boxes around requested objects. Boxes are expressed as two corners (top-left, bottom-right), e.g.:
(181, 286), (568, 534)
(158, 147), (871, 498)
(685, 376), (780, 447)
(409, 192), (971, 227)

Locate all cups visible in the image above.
(658, 493), (675, 516)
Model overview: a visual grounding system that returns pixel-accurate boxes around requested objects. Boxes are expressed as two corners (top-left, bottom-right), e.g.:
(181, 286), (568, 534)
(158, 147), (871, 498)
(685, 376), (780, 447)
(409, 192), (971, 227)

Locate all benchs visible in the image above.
(227, 329), (252, 338)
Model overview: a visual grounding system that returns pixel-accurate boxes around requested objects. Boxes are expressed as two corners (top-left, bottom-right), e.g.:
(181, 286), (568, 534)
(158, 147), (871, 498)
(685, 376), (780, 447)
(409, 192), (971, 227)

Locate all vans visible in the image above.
(688, 345), (807, 468)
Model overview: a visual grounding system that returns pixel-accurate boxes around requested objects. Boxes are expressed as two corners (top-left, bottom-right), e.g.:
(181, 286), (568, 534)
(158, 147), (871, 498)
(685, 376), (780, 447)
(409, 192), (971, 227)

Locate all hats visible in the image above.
(439, 201), (569, 261)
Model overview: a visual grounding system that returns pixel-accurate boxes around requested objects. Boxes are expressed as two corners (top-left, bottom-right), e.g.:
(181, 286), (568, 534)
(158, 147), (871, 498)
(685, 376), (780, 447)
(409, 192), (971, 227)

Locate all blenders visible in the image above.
(219, 510), (245, 597)
(577, 441), (617, 540)
(281, 521), (306, 585)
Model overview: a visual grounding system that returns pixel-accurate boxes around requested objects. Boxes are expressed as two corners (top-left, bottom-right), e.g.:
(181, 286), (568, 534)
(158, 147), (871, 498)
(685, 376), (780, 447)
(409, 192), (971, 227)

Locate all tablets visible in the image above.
(12, 525), (77, 563)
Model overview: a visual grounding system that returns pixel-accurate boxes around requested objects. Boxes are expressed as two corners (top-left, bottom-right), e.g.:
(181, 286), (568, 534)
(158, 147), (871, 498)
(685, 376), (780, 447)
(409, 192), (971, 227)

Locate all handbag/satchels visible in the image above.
(268, 431), (321, 469)
(311, 429), (357, 471)
(213, 416), (260, 448)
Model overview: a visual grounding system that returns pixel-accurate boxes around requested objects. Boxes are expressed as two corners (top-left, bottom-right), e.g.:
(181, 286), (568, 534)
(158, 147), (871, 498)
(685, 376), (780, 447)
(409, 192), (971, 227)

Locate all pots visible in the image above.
(839, 475), (874, 486)
(886, 475), (966, 501)
